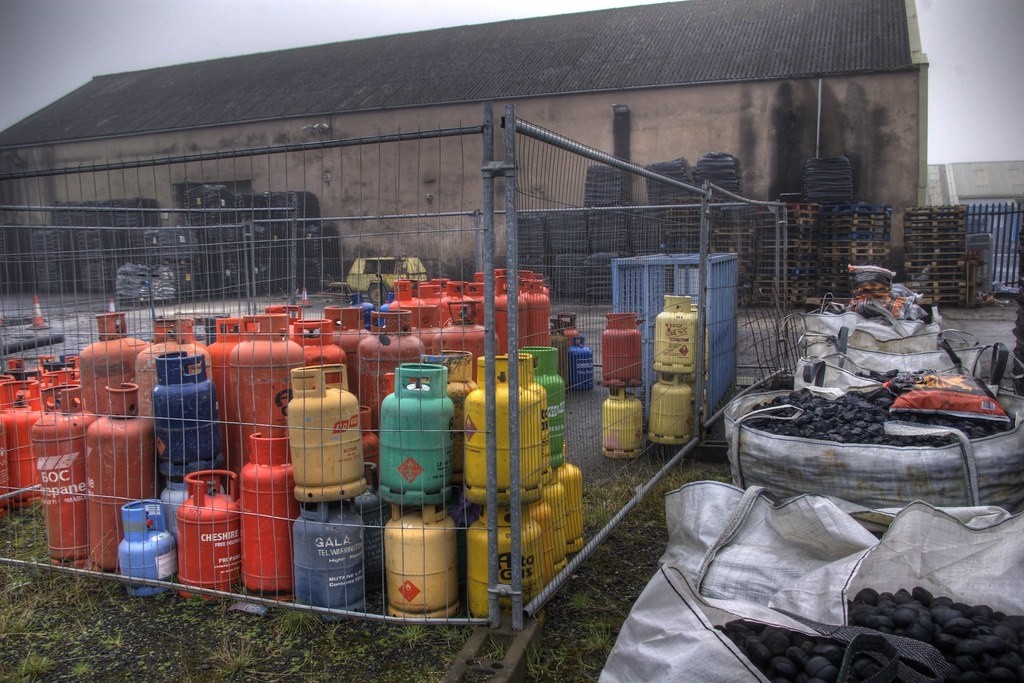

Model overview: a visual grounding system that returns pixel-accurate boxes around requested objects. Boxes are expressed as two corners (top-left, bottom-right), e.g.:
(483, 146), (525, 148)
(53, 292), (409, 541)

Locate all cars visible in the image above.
(347, 255), (428, 304)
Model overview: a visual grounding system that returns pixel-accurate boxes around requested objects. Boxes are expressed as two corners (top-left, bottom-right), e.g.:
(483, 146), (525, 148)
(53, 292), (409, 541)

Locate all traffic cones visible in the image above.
(108, 297), (117, 313)
(27, 294), (53, 330)
(301, 287), (312, 308)
